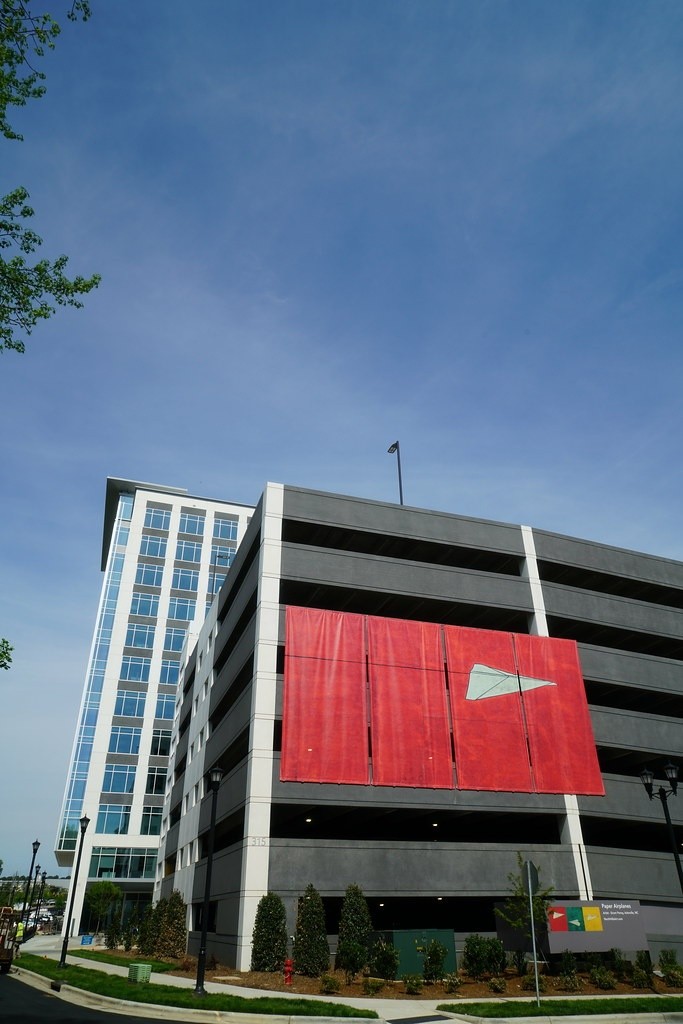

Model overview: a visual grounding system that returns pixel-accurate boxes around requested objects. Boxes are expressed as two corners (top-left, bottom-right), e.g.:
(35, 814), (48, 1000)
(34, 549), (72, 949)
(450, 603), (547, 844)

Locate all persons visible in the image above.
(7, 918), (24, 959)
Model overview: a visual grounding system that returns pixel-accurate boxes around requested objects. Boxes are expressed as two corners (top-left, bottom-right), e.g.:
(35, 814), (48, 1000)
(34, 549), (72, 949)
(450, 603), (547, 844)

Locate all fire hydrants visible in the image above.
(284, 959), (294, 985)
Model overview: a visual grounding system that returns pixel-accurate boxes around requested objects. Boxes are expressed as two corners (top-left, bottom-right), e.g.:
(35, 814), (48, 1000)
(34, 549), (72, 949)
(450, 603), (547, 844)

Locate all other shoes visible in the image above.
(16, 955), (21, 958)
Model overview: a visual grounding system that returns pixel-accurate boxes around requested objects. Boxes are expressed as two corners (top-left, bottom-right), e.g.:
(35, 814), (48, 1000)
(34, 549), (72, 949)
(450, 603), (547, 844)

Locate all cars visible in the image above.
(14, 898), (56, 931)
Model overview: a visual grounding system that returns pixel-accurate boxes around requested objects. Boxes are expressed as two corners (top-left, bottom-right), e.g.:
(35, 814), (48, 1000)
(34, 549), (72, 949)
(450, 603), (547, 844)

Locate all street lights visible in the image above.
(387, 440), (404, 504)
(58, 814), (90, 968)
(193, 763), (224, 995)
(34, 871), (47, 923)
(639, 764), (683, 889)
(17, 838), (20, 924)
(25, 864), (41, 929)
